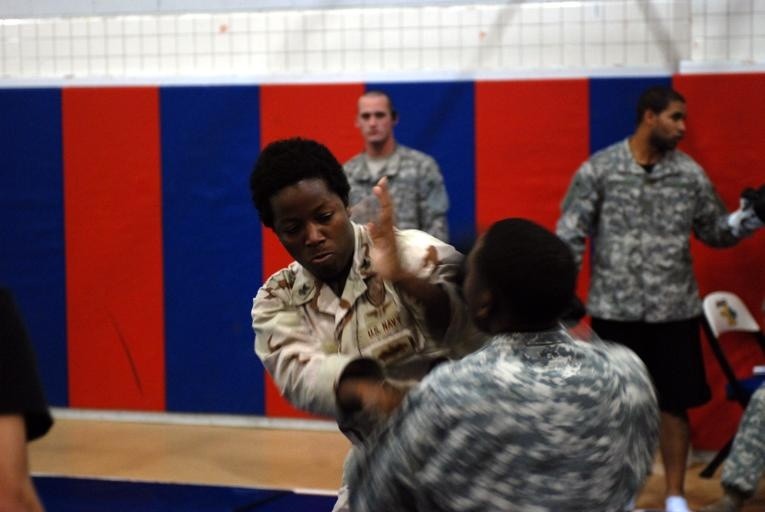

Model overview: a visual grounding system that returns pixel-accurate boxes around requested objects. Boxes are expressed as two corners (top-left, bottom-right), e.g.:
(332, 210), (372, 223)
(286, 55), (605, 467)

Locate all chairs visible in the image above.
(697, 289), (765, 477)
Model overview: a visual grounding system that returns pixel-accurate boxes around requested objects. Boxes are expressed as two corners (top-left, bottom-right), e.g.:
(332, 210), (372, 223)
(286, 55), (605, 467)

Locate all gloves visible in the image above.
(721, 184), (765, 240)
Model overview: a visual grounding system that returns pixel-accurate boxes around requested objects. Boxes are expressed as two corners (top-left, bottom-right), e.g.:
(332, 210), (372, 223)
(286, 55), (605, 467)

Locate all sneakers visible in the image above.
(663, 494), (693, 511)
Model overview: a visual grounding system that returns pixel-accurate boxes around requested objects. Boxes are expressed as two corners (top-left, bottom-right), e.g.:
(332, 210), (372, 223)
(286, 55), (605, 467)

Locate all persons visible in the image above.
(324, 214), (663, 510)
(1, 264), (54, 512)
(704, 385), (765, 511)
(240, 134), (489, 435)
(548, 79), (764, 512)
(337, 89), (450, 240)
